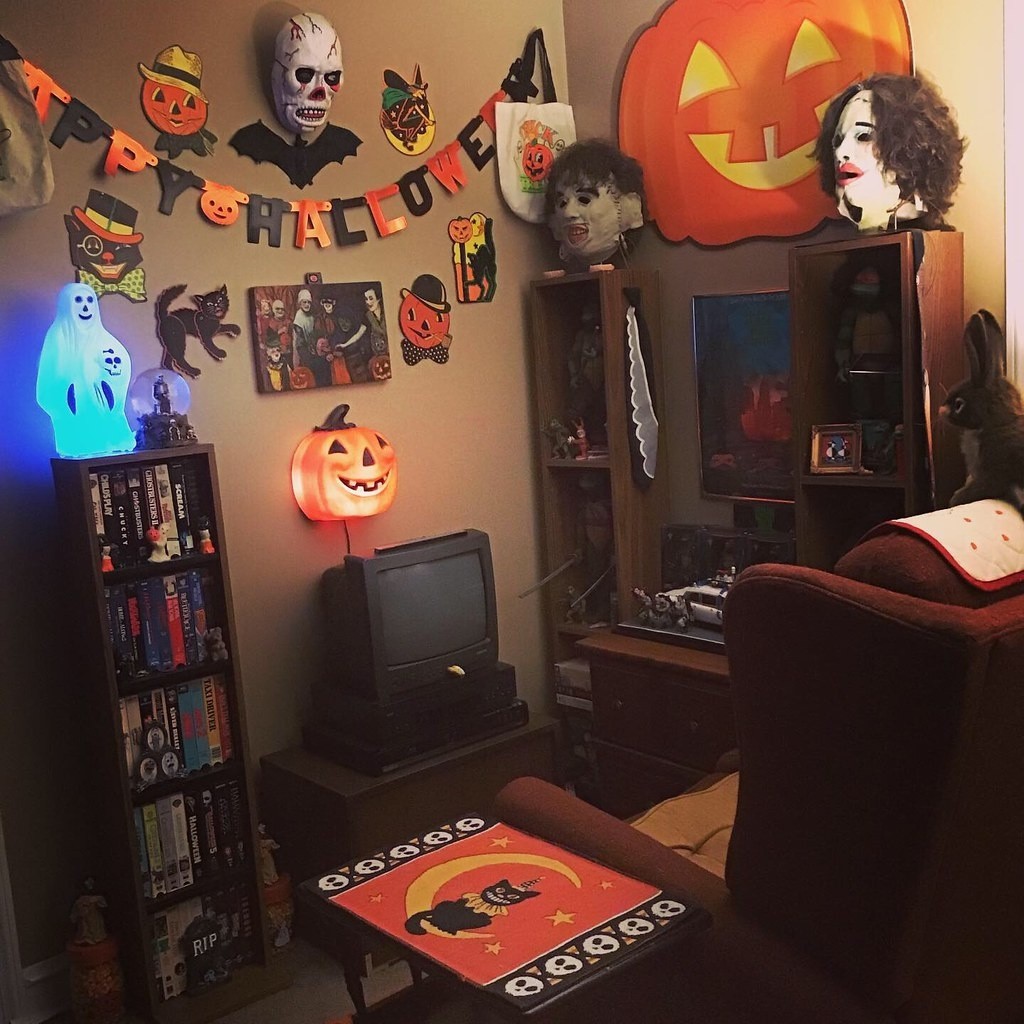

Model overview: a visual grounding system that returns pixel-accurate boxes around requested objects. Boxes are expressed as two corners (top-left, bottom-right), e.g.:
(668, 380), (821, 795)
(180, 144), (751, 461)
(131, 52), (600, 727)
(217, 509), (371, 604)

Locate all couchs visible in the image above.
(493, 498), (1024, 1024)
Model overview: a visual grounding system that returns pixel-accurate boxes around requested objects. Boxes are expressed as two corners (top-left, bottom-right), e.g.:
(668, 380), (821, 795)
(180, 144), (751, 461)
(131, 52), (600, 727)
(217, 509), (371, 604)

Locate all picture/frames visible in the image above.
(811, 424), (864, 474)
(691, 289), (795, 505)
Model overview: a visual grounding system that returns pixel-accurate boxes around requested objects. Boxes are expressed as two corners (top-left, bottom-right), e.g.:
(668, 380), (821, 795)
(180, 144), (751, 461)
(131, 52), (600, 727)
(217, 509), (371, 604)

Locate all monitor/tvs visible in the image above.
(320, 529), (500, 700)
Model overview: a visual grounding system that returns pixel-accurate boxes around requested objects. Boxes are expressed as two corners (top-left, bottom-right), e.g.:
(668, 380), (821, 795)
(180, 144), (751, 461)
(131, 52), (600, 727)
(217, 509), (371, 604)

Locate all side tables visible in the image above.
(297, 812), (713, 1023)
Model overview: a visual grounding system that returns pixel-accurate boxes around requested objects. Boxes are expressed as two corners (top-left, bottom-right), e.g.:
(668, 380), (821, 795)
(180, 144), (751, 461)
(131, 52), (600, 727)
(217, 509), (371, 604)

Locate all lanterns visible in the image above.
(292, 404), (399, 522)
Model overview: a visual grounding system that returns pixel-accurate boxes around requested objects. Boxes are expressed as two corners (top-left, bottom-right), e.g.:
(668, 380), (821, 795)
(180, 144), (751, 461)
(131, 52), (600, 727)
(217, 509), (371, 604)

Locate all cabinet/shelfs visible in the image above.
(788, 233), (965, 573)
(572, 631), (733, 822)
(529, 270), (673, 808)
(51, 443), (293, 1024)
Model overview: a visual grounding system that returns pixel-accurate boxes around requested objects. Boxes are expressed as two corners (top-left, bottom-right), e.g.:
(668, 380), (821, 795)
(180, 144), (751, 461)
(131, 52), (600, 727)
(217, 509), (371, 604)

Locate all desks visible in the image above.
(260, 714), (571, 980)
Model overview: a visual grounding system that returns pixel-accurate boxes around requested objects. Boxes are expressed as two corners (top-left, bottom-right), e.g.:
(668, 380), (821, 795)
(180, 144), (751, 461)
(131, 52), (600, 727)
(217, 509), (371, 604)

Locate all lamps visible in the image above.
(36, 283), (137, 460)
(290, 404), (399, 522)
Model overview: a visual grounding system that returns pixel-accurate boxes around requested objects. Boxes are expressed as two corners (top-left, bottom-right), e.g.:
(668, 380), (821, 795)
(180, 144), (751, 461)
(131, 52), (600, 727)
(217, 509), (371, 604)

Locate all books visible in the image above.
(89, 461), (264, 1000)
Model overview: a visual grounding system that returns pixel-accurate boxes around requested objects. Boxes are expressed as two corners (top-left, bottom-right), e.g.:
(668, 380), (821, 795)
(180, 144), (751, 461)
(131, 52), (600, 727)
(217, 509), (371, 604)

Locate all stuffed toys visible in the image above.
(938, 308), (1023, 505)
